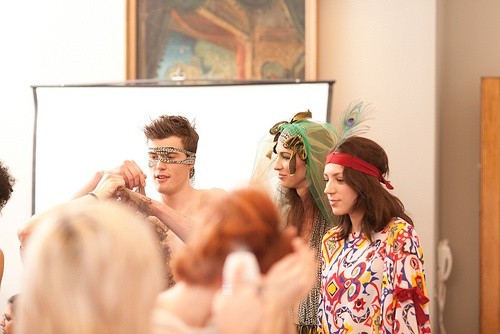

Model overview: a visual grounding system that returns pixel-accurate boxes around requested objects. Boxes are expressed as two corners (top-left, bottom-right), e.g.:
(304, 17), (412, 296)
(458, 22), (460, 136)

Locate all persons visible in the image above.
(266, 110), (338, 334)
(115, 114), (227, 292)
(0, 162), (317, 334)
(317, 100), (432, 334)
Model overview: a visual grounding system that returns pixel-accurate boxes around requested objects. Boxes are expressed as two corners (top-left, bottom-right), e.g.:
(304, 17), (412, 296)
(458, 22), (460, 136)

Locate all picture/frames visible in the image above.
(126, 0), (317, 84)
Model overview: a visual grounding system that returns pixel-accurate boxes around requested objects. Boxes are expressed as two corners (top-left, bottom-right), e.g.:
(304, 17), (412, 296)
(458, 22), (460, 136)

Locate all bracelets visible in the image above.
(87, 192), (98, 199)
